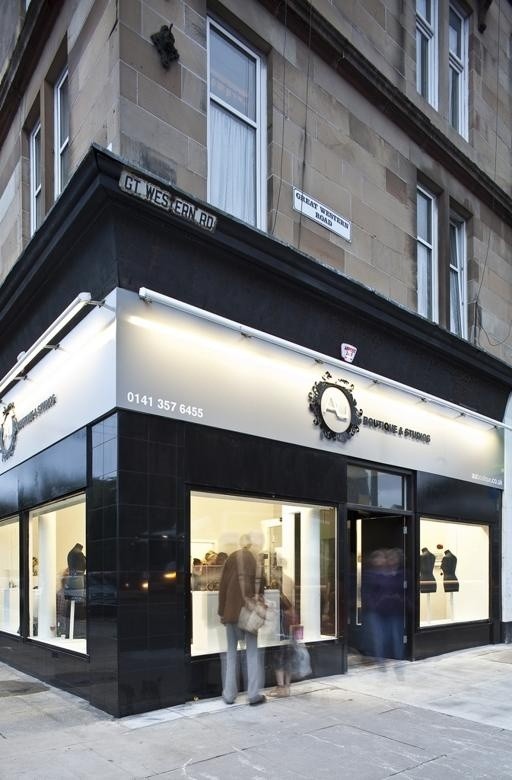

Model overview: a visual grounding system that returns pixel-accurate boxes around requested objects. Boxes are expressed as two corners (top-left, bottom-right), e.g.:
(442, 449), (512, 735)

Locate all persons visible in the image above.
(363, 546), (407, 682)
(440, 549), (459, 592)
(190, 551), (229, 592)
(267, 567), (295, 697)
(67, 542), (88, 590)
(419, 546), (437, 592)
(217, 533), (268, 705)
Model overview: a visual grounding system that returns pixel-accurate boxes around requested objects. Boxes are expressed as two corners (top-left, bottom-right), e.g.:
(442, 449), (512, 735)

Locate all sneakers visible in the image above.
(250, 695), (265, 705)
(224, 696), (235, 704)
(267, 686), (291, 698)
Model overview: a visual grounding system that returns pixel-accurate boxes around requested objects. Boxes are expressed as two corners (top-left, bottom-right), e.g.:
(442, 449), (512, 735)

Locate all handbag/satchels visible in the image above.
(292, 643), (311, 681)
(238, 597), (272, 634)
